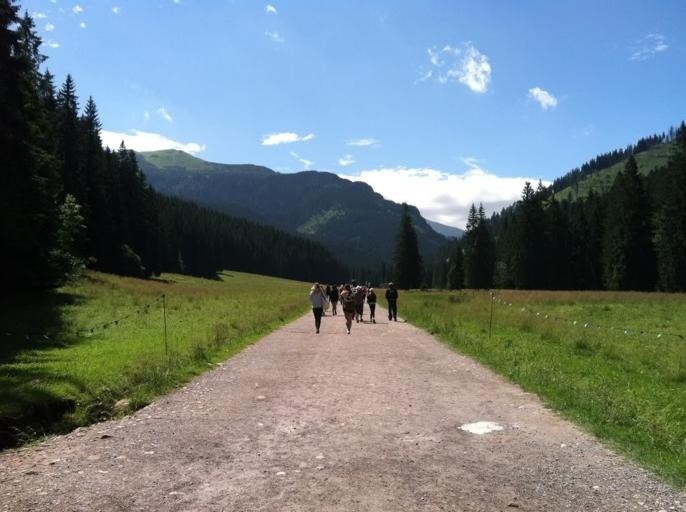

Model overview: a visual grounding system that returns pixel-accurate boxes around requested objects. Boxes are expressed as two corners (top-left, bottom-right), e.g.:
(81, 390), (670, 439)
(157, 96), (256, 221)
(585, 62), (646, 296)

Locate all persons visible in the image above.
(308, 282), (330, 334)
(366, 287), (377, 325)
(385, 281), (398, 322)
(340, 284), (356, 335)
(320, 278), (371, 323)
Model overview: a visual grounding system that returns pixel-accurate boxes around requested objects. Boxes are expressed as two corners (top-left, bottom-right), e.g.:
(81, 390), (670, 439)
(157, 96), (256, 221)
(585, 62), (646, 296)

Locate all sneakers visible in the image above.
(316, 329), (350, 334)
(356, 316), (397, 323)
